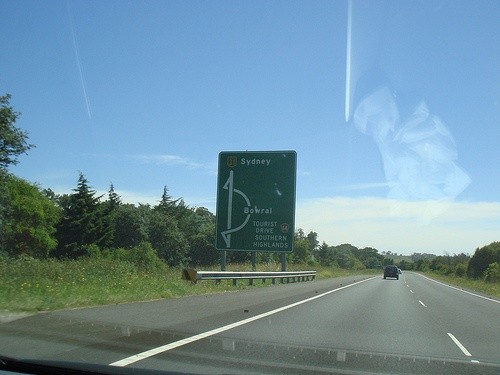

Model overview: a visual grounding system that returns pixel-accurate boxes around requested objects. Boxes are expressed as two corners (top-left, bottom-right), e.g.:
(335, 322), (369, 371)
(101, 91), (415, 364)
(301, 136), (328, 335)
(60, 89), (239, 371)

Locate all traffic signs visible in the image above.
(218, 149), (295, 254)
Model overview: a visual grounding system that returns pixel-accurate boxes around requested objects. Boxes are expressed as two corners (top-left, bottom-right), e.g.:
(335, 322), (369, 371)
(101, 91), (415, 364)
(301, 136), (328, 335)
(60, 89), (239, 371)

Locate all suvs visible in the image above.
(385, 265), (399, 280)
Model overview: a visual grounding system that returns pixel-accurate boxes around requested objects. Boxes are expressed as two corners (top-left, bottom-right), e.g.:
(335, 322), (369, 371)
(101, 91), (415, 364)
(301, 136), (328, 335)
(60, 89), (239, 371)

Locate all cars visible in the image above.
(398, 269), (402, 274)
